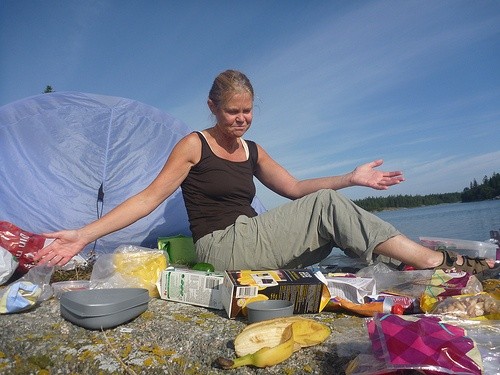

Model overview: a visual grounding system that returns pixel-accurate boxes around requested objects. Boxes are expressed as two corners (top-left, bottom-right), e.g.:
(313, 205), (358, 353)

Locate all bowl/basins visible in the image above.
(245, 300), (295, 323)
(51, 280), (90, 299)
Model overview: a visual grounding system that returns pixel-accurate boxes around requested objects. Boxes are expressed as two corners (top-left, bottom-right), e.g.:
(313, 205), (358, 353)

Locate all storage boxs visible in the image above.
(419, 236), (499, 260)
(60, 289), (150, 329)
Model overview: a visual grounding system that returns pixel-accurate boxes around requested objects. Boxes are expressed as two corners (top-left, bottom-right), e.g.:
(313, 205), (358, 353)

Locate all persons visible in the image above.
(34, 69), (500, 281)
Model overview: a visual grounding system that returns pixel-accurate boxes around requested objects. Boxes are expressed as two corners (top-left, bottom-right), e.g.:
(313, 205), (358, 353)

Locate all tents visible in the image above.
(0, 91), (267, 261)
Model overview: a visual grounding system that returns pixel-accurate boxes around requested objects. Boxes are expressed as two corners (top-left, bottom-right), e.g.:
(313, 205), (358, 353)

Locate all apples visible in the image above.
(390, 304), (404, 315)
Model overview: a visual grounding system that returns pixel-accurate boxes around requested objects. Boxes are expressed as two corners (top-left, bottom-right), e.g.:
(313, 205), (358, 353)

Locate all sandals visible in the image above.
(424, 248), (500, 281)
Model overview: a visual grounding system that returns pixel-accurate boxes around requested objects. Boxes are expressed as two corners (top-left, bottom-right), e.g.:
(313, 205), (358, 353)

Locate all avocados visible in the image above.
(191, 263), (214, 272)
